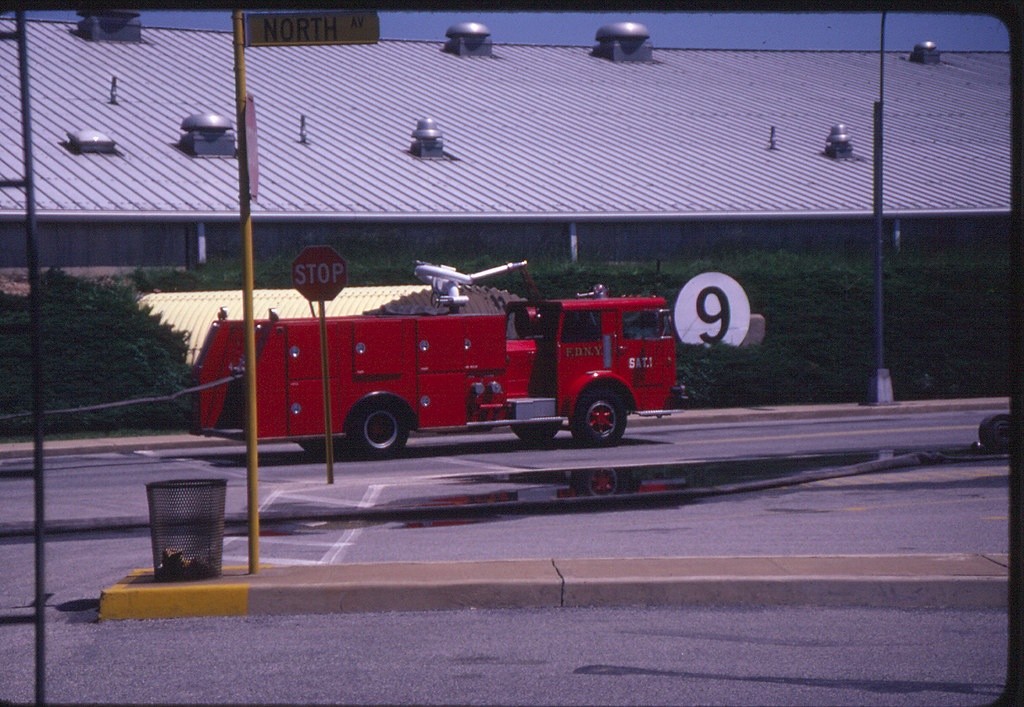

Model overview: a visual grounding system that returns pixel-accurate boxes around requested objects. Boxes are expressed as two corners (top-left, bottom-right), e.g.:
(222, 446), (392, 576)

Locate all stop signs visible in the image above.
(291, 244), (346, 302)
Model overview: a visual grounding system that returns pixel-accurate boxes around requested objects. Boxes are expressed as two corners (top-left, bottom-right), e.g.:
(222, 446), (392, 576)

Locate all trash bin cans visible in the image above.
(146, 480), (227, 582)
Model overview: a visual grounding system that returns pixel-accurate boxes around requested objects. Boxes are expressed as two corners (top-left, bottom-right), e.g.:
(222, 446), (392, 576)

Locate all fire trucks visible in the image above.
(180, 258), (686, 457)
